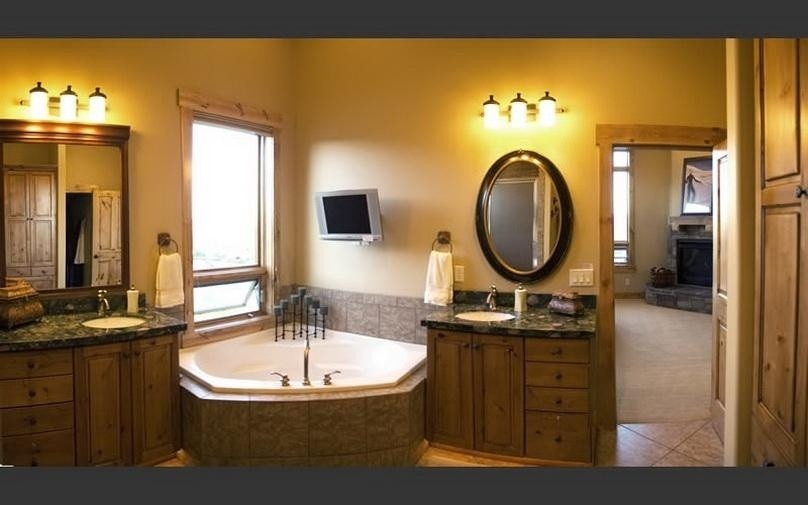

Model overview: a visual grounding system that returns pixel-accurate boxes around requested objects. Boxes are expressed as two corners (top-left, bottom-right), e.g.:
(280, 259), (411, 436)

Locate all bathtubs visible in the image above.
(178, 322), (428, 395)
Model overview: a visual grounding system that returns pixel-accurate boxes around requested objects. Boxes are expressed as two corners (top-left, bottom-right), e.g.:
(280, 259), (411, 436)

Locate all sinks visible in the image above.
(455, 311), (515, 322)
(80, 315), (146, 329)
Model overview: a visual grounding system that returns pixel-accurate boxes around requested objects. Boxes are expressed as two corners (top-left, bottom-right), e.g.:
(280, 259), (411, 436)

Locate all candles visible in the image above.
(271, 285), (329, 318)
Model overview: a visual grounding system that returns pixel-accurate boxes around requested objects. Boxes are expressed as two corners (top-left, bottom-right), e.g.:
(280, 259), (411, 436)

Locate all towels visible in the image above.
(423, 249), (455, 308)
(154, 252), (184, 308)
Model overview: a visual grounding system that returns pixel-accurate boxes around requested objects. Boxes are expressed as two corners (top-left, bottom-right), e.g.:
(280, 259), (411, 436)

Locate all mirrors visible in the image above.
(0, 118), (131, 297)
(473, 147), (571, 285)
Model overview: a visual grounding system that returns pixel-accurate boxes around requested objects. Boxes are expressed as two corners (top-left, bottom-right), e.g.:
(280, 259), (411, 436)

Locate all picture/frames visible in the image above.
(678, 155), (714, 216)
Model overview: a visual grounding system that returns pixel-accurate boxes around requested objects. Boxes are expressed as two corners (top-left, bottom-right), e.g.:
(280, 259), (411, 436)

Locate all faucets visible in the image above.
(97, 289), (111, 313)
(301, 339), (313, 385)
(484, 284), (496, 310)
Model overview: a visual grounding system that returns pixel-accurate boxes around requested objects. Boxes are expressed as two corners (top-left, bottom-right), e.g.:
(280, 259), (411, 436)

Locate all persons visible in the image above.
(685, 169), (702, 204)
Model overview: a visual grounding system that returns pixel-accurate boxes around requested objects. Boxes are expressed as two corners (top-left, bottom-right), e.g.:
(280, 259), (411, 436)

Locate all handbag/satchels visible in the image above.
(649, 265), (676, 289)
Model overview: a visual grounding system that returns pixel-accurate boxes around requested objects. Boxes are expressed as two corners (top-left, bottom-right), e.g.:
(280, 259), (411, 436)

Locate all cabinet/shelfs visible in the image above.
(522, 336), (603, 466)
(73, 331), (181, 467)
(741, 37), (807, 471)
(1, 162), (58, 290)
(0, 346), (76, 471)
(423, 329), (524, 468)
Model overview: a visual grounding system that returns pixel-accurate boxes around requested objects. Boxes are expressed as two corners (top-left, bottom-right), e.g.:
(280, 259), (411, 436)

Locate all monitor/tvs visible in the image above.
(315, 188), (383, 240)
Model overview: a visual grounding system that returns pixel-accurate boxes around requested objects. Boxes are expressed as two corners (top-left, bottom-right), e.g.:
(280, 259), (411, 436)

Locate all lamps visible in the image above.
(479, 91), (564, 128)
(17, 82), (110, 123)
